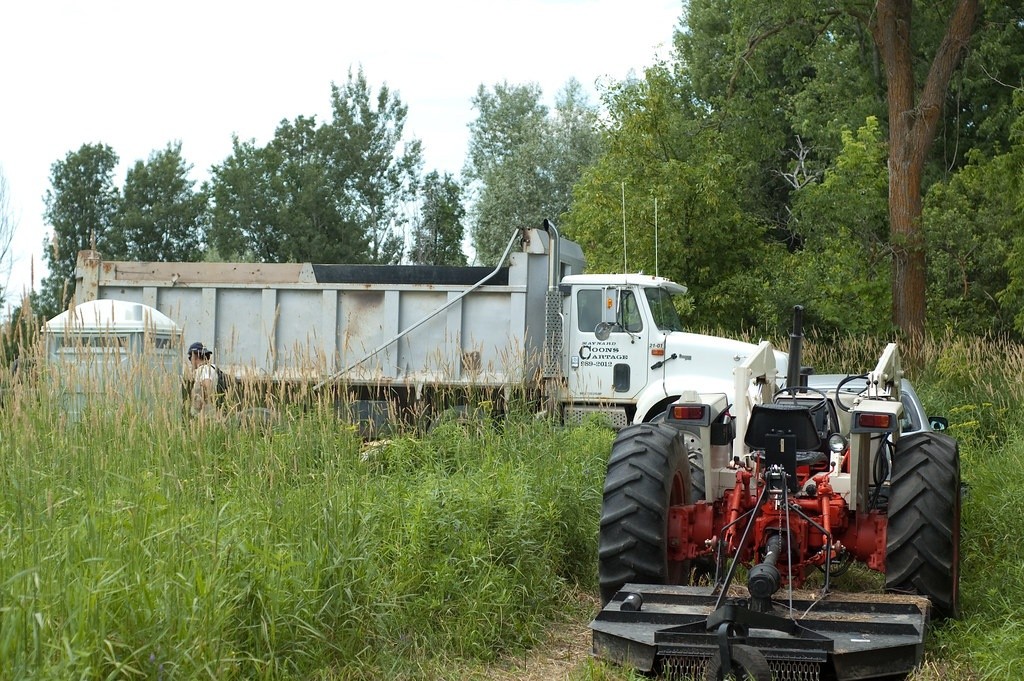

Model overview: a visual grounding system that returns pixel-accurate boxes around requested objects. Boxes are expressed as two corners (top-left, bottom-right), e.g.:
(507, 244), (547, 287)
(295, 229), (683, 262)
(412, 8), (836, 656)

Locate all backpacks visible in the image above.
(210, 365), (229, 407)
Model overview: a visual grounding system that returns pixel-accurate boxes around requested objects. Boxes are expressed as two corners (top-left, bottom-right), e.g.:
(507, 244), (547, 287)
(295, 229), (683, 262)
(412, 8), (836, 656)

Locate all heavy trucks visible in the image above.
(71, 218), (789, 446)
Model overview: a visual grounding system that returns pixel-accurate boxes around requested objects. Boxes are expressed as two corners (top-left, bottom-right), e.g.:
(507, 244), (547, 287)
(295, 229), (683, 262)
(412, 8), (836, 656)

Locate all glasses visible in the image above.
(188, 354), (194, 361)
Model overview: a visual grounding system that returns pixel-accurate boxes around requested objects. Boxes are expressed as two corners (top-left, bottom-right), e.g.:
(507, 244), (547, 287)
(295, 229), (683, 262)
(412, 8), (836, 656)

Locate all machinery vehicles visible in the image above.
(590, 296), (960, 680)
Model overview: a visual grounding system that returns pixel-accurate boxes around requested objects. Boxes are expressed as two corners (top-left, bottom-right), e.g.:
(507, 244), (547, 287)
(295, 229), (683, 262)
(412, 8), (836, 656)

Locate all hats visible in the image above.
(188, 342), (212, 355)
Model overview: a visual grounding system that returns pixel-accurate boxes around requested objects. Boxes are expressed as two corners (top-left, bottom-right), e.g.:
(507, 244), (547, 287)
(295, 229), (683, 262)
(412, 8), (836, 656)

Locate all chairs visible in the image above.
(744, 404), (826, 464)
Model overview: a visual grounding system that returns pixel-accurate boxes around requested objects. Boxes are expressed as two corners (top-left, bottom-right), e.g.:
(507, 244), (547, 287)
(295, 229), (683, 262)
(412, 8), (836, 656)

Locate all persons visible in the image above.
(188, 343), (217, 425)
